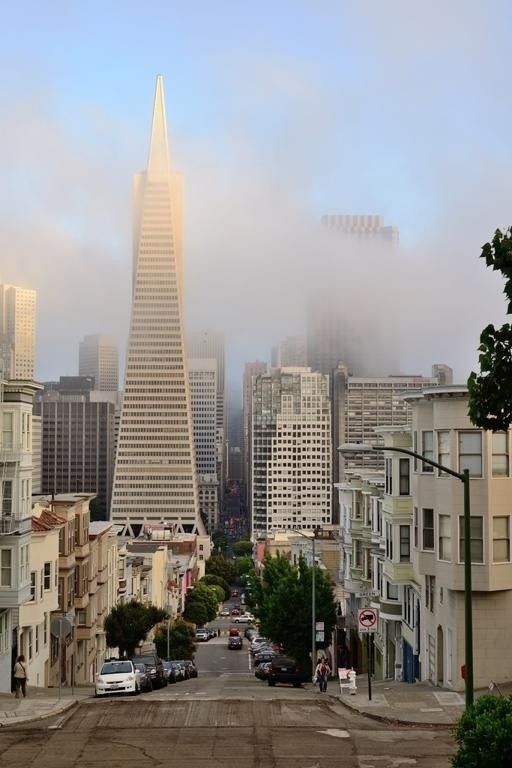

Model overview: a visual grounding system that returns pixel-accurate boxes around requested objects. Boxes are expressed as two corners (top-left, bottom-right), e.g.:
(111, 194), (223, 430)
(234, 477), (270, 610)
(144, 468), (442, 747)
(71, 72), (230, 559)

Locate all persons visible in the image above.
(13, 655), (30, 698)
(314, 658), (331, 693)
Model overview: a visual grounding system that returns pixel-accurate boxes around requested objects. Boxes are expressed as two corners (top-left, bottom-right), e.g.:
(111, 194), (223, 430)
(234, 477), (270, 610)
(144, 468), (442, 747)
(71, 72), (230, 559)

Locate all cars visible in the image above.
(232, 607), (240, 615)
(195, 628), (216, 641)
(224, 521), (236, 539)
(229, 636), (242, 649)
(220, 608), (230, 616)
(232, 556), (236, 560)
(232, 615), (254, 624)
(245, 627), (303, 686)
(95, 654), (197, 697)
(228, 627), (239, 636)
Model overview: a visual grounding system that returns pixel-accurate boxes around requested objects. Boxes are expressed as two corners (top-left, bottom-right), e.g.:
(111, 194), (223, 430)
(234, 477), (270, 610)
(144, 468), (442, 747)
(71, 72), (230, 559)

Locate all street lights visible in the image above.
(271, 528), (316, 675)
(336, 442), (474, 710)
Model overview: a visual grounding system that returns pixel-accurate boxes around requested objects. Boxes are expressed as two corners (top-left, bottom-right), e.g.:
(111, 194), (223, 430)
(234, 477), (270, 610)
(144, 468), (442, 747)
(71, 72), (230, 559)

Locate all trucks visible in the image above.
(232, 588), (238, 597)
